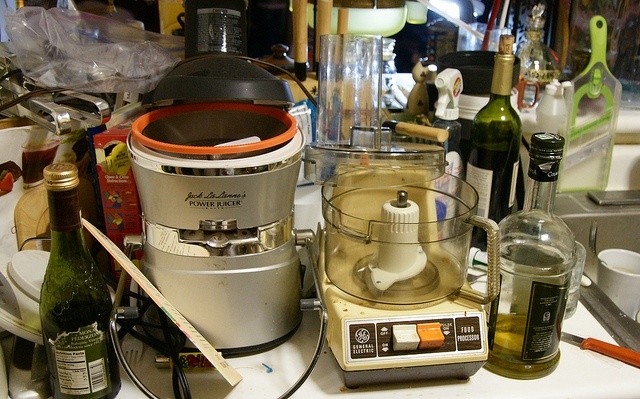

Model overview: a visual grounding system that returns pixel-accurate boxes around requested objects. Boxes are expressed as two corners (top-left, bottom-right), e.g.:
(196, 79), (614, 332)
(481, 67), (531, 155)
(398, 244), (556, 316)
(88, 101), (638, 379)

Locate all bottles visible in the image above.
(486, 133), (578, 381)
(38, 162), (122, 397)
(464, 33), (523, 247)
(517, 3), (564, 110)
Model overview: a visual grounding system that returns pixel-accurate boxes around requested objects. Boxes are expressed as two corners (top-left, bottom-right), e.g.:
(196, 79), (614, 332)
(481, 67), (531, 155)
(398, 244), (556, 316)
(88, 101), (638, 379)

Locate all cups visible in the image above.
(593, 247), (638, 325)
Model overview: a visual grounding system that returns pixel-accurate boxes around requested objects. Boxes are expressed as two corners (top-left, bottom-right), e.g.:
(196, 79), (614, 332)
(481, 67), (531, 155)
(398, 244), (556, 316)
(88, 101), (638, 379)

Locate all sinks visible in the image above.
(550, 191), (639, 350)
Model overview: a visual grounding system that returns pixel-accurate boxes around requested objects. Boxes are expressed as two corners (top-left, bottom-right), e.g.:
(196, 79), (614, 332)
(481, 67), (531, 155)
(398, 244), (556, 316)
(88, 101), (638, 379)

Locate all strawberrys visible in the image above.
(0, 169), (14, 192)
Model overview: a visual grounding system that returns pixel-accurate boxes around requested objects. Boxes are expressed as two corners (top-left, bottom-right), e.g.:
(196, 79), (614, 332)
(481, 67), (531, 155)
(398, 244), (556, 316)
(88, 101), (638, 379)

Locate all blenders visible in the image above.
(309, 161), (488, 389)
